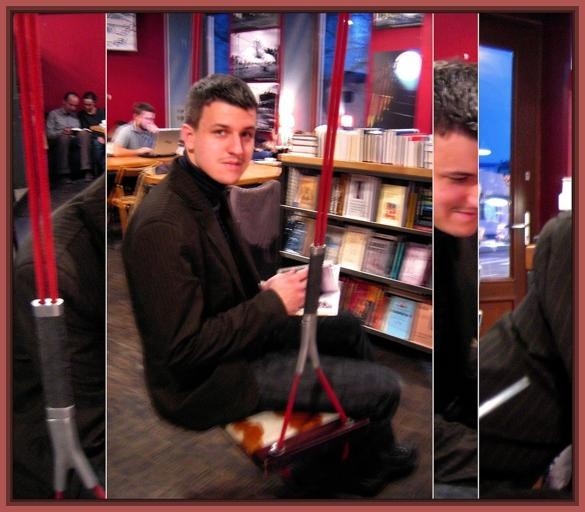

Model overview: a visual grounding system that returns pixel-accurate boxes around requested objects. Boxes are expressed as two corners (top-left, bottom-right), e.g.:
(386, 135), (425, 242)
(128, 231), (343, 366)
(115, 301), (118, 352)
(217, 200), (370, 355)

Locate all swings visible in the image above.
(188, 8), (372, 468)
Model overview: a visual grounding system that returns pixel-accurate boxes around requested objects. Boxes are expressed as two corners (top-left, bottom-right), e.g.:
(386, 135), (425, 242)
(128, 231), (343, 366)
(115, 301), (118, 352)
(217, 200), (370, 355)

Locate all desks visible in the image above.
(106, 156), (181, 238)
(126, 158), (281, 235)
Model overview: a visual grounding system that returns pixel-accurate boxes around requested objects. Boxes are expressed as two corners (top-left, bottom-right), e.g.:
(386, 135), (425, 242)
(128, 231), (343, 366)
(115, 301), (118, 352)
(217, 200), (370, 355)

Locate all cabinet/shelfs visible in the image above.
(278, 153), (432, 353)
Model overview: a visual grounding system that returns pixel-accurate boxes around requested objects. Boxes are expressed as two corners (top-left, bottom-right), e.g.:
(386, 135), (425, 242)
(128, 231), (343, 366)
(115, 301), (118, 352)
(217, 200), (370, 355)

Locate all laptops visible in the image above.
(139, 129), (181, 158)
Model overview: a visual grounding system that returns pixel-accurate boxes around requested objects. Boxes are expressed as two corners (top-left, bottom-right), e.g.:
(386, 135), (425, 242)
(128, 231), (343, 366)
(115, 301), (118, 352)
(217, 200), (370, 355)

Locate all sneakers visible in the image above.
(84, 172), (94, 182)
(62, 176), (72, 184)
(373, 439), (421, 479)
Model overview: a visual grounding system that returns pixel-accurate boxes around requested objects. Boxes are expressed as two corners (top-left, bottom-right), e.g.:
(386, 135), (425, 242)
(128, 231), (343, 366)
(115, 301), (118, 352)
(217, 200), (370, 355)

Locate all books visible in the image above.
(281, 124), (432, 348)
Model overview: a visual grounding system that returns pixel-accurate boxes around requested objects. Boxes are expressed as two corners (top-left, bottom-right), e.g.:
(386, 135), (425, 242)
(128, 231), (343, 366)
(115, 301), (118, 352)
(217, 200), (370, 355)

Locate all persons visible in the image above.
(45, 91), (93, 185)
(13, 167), (106, 499)
(480, 205), (579, 498)
(434, 59), (478, 499)
(120, 73), (424, 495)
(77, 91), (105, 171)
(107, 102), (159, 157)
